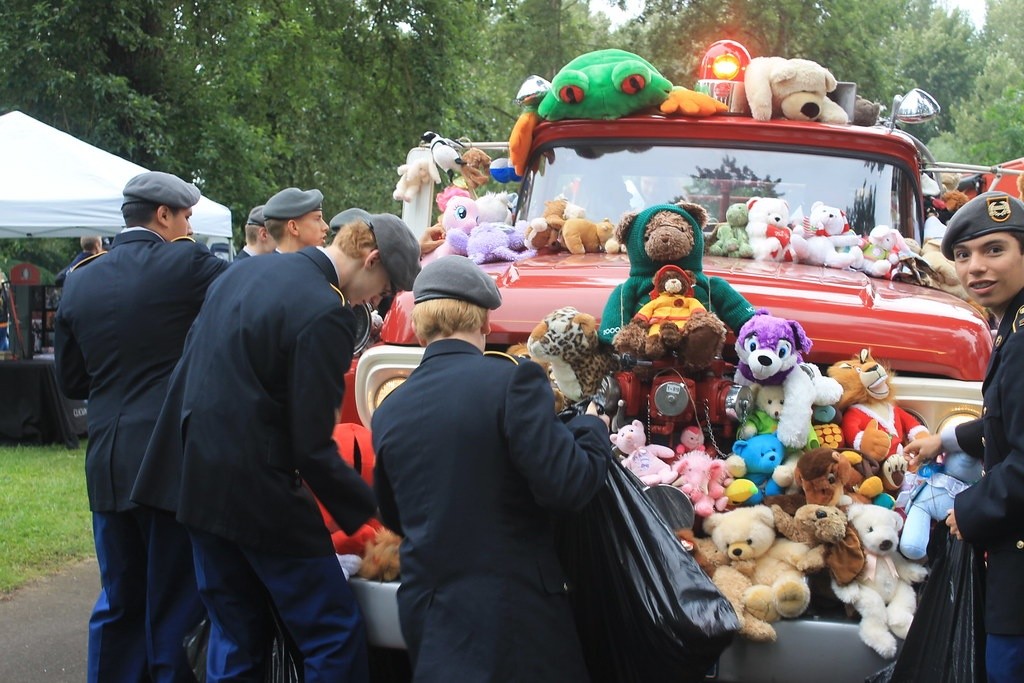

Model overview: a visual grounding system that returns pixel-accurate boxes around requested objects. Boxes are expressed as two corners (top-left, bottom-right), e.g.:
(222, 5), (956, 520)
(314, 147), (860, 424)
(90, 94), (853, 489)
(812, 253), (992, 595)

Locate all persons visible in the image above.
(904, 192), (1024, 683)
(53, 167), (422, 683)
(370, 254), (614, 683)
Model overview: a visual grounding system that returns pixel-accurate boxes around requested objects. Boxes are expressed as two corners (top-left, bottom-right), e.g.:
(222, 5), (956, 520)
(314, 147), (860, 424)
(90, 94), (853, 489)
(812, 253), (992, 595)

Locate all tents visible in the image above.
(0, 110), (235, 261)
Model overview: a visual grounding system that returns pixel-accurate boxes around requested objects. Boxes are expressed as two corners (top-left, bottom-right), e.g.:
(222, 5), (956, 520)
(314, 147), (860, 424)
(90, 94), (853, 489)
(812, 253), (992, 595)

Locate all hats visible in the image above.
(263, 187), (324, 221)
(362, 212), (422, 291)
(329, 208), (370, 235)
(246, 205), (265, 226)
(121, 171), (201, 210)
(413, 255), (503, 310)
(941, 190), (1024, 261)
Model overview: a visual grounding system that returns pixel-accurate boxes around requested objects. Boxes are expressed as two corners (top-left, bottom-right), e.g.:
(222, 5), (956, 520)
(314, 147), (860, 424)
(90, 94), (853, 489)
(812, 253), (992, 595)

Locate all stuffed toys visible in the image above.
(304, 32), (989, 659)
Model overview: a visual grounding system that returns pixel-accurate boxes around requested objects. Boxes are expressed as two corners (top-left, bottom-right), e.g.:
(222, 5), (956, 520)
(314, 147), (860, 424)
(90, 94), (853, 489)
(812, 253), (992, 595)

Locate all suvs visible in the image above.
(315, 40), (996, 683)
